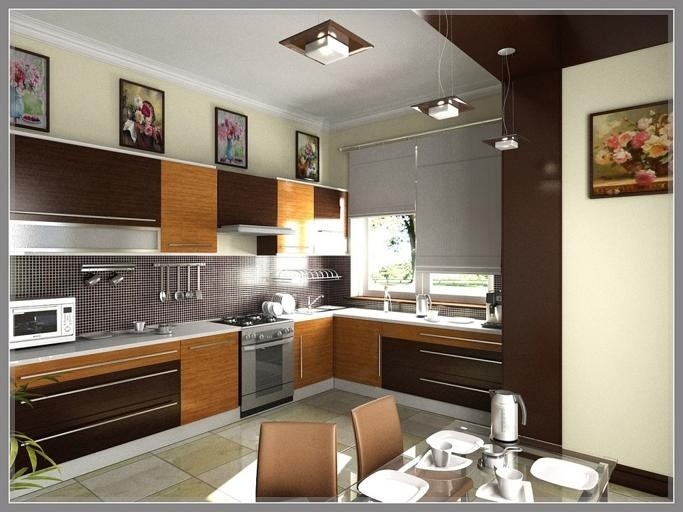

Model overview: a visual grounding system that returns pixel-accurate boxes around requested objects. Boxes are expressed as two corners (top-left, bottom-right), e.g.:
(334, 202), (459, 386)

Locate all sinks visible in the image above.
(315, 304), (346, 309)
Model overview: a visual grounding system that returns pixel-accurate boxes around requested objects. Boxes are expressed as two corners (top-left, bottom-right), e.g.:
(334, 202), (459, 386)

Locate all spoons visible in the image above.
(159, 265), (166, 303)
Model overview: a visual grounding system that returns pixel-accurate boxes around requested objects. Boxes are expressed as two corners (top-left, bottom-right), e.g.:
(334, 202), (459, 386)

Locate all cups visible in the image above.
(481, 443), (505, 469)
(131, 320), (145, 332)
(85, 275), (100, 287)
(109, 275), (122, 287)
(494, 467), (523, 498)
(158, 323), (169, 331)
(429, 440), (453, 466)
(426, 311), (438, 319)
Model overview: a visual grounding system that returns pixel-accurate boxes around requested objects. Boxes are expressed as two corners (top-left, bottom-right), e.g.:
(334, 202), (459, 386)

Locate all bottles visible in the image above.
(382, 286), (392, 315)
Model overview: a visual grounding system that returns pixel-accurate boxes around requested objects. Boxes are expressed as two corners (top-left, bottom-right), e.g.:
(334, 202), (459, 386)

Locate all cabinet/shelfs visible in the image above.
(333, 317), (380, 389)
(10, 340), (182, 476)
(312, 182), (349, 256)
(380, 322), (502, 409)
(177, 333), (239, 425)
(8, 128), (158, 256)
(160, 159), (218, 253)
(291, 319), (334, 387)
(218, 170), (310, 257)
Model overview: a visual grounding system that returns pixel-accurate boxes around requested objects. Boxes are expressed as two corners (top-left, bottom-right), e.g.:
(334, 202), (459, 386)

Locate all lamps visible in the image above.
(411, 10), (474, 125)
(483, 49), (533, 152)
(278, 8), (373, 67)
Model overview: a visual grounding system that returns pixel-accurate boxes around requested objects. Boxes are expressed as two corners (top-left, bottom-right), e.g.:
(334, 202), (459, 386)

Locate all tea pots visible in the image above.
(414, 294), (431, 318)
(487, 388), (526, 442)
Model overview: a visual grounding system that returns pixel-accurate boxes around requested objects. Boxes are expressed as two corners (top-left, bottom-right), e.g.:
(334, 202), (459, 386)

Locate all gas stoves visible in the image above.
(212, 312), (290, 327)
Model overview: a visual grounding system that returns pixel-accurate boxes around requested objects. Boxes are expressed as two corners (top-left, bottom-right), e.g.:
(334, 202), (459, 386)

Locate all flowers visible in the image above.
(220, 118), (244, 140)
(302, 143), (317, 161)
(9, 58), (40, 95)
(597, 108), (671, 187)
(127, 92), (157, 138)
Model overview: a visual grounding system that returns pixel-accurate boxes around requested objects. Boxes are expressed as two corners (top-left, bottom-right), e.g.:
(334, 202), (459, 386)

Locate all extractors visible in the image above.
(218, 223), (295, 239)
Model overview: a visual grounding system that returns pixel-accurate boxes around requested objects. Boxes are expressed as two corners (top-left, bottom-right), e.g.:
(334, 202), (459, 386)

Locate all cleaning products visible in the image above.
(383, 285), (392, 314)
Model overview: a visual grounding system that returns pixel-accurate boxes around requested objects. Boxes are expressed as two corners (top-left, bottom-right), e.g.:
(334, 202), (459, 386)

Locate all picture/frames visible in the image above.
(7, 43), (51, 133)
(212, 106), (249, 171)
(586, 99), (673, 201)
(294, 130), (320, 183)
(116, 77), (167, 156)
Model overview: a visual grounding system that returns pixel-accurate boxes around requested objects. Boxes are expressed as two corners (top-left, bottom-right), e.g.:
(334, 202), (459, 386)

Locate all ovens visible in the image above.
(236, 322), (294, 417)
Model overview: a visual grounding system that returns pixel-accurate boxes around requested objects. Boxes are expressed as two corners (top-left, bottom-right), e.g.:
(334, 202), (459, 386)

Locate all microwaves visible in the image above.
(6, 295), (77, 351)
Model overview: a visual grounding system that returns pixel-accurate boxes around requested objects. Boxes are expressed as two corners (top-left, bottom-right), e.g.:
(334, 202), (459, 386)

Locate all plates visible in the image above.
(415, 449), (471, 470)
(530, 457), (599, 491)
(151, 331), (172, 334)
(358, 468), (427, 502)
(78, 332), (113, 340)
(448, 317), (473, 323)
(425, 429), (485, 454)
(475, 480), (533, 503)
(22, 115), (40, 124)
(261, 290), (295, 319)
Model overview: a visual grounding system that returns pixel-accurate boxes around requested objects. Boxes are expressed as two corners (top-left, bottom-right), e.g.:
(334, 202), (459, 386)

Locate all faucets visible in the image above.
(307, 293), (325, 309)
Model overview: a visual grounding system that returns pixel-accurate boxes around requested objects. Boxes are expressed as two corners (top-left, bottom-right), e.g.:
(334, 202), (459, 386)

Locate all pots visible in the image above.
(480, 445), (522, 467)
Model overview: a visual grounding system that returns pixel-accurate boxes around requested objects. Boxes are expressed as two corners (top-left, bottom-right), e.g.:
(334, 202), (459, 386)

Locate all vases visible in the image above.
(224, 137), (234, 160)
(622, 150), (668, 181)
(10, 85), (23, 118)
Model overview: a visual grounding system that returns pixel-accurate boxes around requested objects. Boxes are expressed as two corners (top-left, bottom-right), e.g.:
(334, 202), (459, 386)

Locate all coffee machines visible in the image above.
(484, 291), (502, 326)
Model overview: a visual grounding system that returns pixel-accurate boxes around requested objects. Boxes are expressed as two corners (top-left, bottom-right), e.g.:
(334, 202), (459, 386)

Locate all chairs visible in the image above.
(348, 387), (473, 503)
(253, 419), (339, 499)
